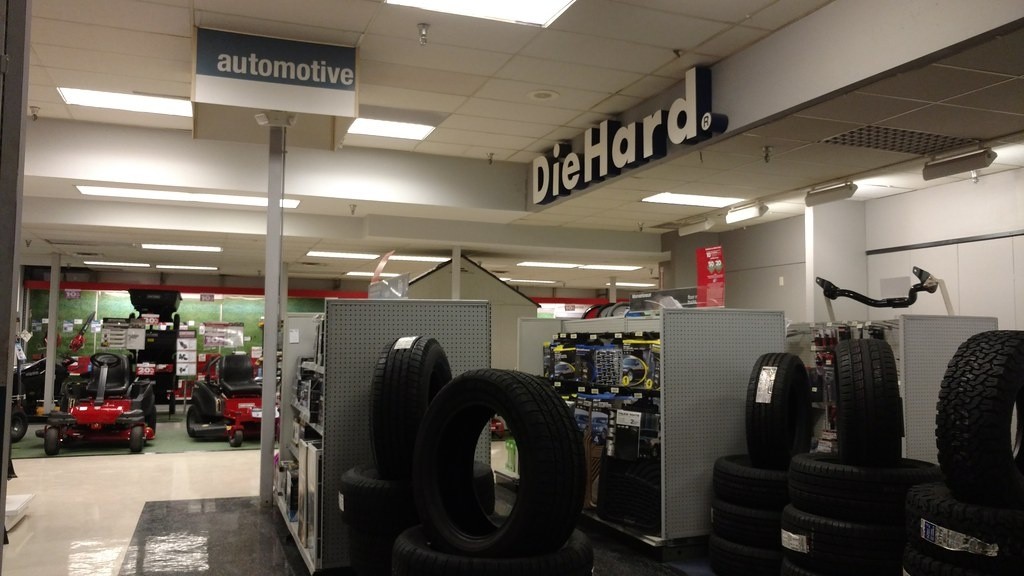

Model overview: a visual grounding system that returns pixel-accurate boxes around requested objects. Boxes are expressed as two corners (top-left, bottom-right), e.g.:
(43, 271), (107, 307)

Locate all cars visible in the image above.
(186, 352), (285, 447)
(35, 352), (158, 457)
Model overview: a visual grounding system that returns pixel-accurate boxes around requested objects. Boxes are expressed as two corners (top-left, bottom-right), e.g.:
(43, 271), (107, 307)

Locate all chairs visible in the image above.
(85, 353), (131, 395)
(221, 355), (262, 394)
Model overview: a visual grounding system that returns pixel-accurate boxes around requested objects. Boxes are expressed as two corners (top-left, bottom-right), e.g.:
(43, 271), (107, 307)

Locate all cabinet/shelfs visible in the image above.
(517, 308), (784, 562)
(271, 297), (491, 576)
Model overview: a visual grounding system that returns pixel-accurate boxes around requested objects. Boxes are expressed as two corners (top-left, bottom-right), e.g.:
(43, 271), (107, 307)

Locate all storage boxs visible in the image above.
(543, 343), (661, 461)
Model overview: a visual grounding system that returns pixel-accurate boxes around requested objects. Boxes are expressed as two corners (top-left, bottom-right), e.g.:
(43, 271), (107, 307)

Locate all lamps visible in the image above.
(726, 199), (769, 224)
(924, 142), (998, 181)
(255, 111), (272, 125)
(678, 217), (716, 237)
(288, 111), (298, 126)
(804, 180), (858, 206)
